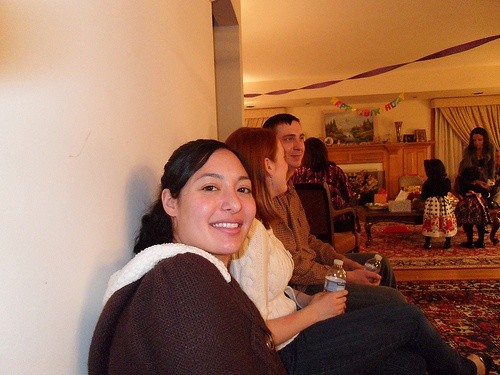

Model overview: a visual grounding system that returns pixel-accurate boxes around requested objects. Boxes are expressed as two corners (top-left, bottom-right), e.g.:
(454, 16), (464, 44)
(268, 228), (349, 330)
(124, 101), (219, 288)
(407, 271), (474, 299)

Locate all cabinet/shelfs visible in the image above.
(326, 141), (434, 200)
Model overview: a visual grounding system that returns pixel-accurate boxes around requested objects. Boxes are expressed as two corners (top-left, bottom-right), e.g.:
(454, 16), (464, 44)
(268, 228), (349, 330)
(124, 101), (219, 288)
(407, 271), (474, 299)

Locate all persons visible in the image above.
(226, 113), (500, 375)
(86, 139), (290, 375)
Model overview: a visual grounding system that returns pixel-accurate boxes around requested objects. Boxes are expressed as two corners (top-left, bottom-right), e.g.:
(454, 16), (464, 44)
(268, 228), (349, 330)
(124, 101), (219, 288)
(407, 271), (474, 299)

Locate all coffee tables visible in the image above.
(364, 206), (500, 248)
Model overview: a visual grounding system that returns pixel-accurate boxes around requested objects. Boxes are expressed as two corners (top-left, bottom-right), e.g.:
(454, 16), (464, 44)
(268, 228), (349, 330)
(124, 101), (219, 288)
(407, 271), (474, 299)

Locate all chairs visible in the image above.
(294, 182), (359, 254)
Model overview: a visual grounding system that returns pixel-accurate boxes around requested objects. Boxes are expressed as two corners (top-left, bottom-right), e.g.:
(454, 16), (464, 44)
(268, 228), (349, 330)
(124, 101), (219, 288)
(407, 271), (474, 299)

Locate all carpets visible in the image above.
(355, 222), (500, 271)
(396, 278), (500, 375)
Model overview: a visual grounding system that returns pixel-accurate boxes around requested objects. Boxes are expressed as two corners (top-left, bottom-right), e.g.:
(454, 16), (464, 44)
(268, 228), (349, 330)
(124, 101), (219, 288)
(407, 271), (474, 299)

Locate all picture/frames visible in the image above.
(322, 108), (377, 143)
(415, 129), (427, 142)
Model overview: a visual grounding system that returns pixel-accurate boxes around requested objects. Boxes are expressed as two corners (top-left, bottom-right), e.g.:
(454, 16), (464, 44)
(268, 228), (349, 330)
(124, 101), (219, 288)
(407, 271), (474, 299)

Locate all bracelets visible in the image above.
(295, 291), (301, 299)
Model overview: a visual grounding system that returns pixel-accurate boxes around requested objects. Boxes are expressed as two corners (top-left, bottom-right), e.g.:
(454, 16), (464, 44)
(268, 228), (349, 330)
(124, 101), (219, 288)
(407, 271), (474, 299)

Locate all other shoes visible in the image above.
(472, 241), (485, 248)
(425, 241), (431, 248)
(444, 242), (450, 248)
(464, 353), (491, 375)
(461, 241), (474, 248)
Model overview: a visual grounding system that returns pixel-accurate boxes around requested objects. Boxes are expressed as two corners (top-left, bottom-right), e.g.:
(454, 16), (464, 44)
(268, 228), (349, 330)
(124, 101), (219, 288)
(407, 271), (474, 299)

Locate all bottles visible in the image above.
(363, 254), (382, 282)
(324, 259), (346, 291)
